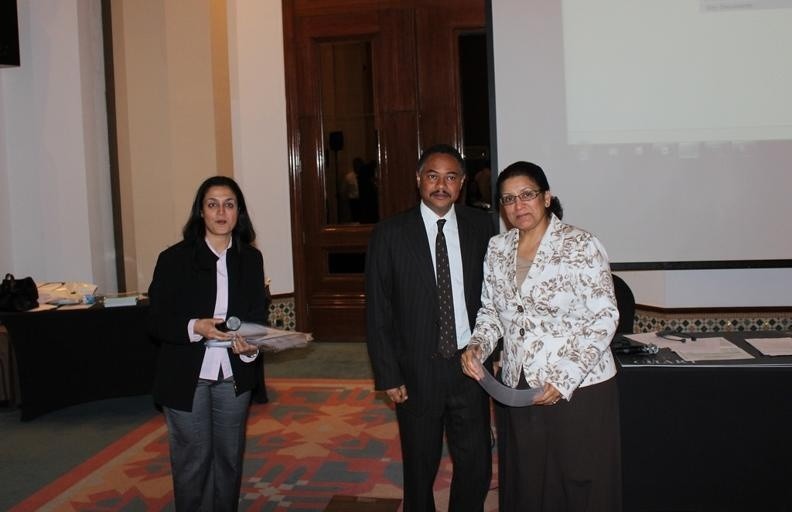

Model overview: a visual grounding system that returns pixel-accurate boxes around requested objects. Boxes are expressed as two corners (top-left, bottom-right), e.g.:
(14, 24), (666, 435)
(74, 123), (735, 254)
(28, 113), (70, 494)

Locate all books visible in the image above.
(204, 322), (315, 354)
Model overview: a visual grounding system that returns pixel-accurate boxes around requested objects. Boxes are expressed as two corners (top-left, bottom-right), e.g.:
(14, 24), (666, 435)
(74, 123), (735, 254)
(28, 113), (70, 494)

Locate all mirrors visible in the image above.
(309, 29), (384, 236)
(448, 21), (494, 216)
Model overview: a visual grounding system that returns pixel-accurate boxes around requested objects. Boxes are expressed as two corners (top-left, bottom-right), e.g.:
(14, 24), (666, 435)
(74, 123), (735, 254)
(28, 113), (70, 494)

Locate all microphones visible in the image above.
(214, 316), (241, 332)
(656, 330), (696, 343)
(612, 343), (659, 355)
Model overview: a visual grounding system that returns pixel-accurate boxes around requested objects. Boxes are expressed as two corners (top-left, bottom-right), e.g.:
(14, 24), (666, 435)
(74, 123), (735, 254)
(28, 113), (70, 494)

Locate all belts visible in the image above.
(430, 352), (455, 361)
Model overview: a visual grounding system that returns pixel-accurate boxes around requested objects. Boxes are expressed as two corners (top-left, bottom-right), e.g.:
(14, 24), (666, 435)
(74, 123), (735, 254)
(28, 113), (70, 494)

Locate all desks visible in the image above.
(0, 292), (155, 422)
(612, 330), (792, 511)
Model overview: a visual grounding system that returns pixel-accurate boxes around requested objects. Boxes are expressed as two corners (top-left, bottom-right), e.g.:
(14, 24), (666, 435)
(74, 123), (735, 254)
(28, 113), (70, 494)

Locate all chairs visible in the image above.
(611, 273), (636, 335)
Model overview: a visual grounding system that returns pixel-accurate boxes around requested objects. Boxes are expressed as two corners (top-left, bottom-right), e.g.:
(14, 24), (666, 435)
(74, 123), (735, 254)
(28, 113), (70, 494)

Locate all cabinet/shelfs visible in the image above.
(281, 1), (500, 343)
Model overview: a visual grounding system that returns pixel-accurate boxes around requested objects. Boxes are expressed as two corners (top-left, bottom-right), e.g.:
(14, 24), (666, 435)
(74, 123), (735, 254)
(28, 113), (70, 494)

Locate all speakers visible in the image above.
(0, 0), (20, 66)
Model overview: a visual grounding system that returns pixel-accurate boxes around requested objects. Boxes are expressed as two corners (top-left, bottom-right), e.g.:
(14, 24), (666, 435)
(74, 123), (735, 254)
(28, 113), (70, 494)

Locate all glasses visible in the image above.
(499, 189), (545, 206)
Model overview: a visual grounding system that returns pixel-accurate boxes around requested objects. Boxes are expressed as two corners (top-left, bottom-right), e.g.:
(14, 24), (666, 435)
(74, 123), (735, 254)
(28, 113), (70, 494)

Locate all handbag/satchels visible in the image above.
(0, 273), (39, 312)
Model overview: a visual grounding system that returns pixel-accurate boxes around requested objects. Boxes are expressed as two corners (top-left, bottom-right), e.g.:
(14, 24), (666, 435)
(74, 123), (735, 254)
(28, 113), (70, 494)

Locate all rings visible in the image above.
(551, 400), (557, 405)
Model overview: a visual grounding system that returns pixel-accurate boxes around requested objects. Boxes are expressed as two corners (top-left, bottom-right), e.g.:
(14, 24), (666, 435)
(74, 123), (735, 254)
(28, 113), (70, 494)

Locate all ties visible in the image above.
(437, 219), (458, 359)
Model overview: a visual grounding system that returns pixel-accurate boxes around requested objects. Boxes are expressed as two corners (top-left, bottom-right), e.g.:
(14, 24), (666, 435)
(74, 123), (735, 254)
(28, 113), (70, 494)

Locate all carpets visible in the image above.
(8, 377), (499, 512)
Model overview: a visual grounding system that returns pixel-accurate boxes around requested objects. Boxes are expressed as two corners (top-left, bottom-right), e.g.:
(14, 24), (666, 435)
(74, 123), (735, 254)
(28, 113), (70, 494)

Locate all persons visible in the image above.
(361, 144), (499, 511)
(145, 176), (269, 511)
(460, 161), (626, 511)
(340, 155), (361, 219)
